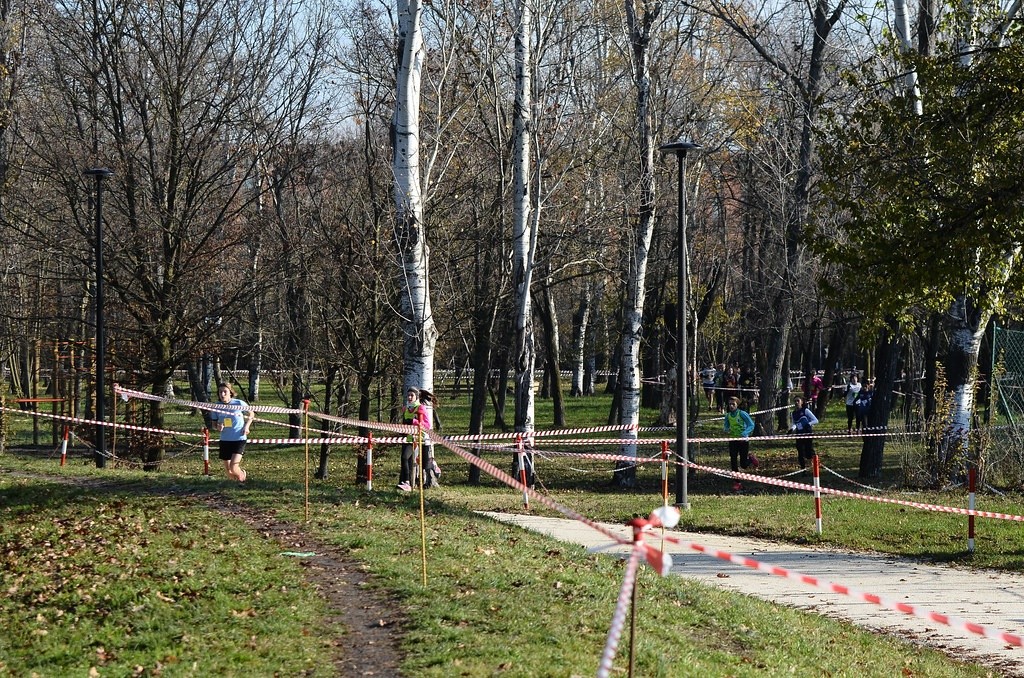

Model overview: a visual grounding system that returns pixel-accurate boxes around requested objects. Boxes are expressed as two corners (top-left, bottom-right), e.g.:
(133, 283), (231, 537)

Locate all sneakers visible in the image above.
(731, 482), (742, 491)
(397, 484), (411, 492)
(749, 453), (758, 466)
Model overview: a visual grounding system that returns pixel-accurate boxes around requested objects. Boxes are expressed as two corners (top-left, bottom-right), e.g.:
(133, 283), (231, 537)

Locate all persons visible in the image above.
(685, 362), (877, 438)
(396, 387), (442, 491)
(722, 396), (759, 491)
(786, 395), (820, 478)
(212, 381), (254, 487)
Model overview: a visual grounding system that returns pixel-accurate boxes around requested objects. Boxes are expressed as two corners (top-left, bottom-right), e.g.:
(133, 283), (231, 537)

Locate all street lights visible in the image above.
(660, 143), (704, 512)
(86, 168), (114, 470)
